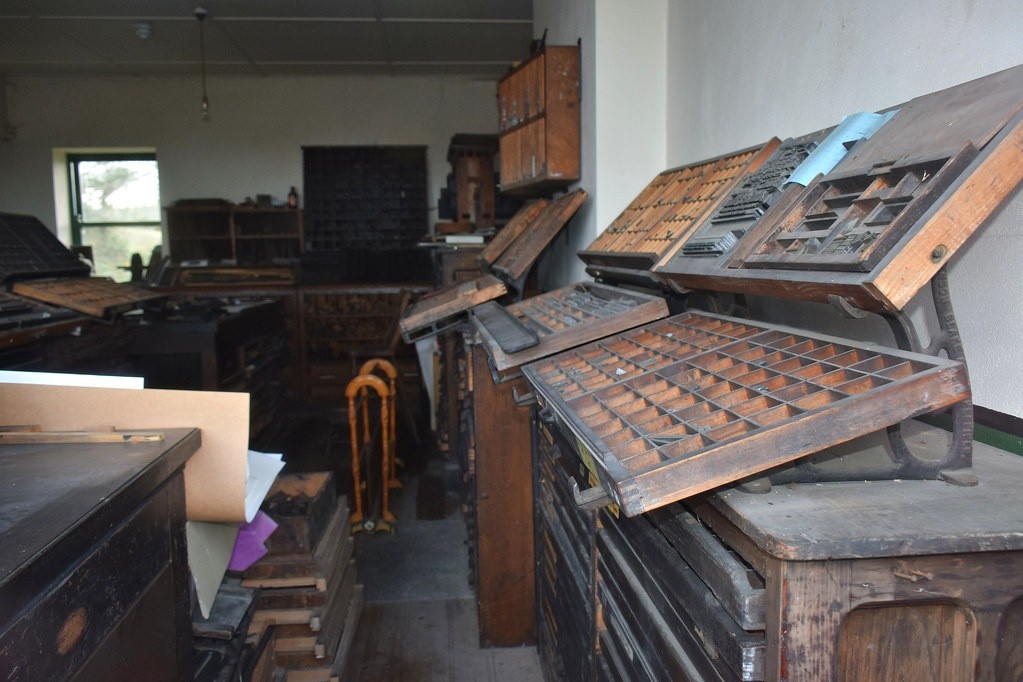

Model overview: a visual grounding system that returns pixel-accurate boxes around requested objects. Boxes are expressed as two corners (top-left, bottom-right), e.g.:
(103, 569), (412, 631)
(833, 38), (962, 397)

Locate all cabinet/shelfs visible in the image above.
(0, 24), (1023, 682)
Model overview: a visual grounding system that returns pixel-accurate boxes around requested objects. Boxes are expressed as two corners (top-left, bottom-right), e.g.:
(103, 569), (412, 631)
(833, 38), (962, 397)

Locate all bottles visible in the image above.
(289, 187), (297, 208)
